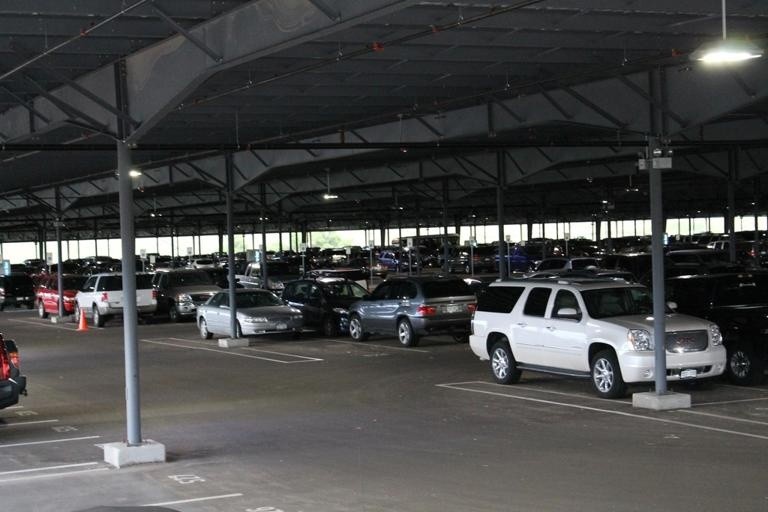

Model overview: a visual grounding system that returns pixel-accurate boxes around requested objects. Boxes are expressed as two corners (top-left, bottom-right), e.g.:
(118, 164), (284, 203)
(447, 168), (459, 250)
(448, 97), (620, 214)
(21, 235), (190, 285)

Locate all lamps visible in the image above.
(689, 0), (763, 61)
(151, 193), (162, 216)
(324, 168), (338, 198)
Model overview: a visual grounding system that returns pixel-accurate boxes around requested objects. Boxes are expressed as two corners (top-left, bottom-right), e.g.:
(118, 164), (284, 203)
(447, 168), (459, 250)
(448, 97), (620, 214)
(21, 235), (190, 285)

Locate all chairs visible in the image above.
(557, 295), (578, 311)
(599, 294), (624, 315)
